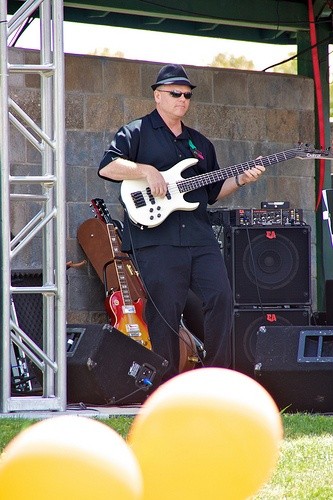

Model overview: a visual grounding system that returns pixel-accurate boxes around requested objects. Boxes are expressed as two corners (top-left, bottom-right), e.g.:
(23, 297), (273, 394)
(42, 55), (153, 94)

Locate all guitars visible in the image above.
(103, 222), (152, 350)
(121, 141), (330, 229)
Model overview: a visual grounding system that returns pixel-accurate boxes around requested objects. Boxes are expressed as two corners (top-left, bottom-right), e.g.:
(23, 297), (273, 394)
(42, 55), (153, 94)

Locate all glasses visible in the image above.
(157, 89), (193, 99)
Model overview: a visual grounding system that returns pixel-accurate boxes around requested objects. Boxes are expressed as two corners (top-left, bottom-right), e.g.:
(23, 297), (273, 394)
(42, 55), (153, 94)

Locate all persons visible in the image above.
(97, 64), (265, 405)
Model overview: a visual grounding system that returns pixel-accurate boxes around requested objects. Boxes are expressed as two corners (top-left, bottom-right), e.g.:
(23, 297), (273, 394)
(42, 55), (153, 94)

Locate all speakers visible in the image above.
(253, 326), (333, 413)
(28, 323), (168, 406)
(224, 225), (312, 306)
(230, 308), (311, 379)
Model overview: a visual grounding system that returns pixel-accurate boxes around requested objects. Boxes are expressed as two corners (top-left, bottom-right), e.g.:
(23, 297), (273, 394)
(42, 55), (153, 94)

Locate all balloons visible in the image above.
(0, 414), (143, 500)
(127, 367), (283, 500)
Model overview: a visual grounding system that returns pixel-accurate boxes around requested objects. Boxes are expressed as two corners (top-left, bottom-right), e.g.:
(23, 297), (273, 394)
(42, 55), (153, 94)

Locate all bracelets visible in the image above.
(235, 175), (246, 188)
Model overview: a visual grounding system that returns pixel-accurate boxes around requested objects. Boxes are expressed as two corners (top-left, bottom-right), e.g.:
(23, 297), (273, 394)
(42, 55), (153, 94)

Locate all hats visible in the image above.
(151, 64), (197, 91)
